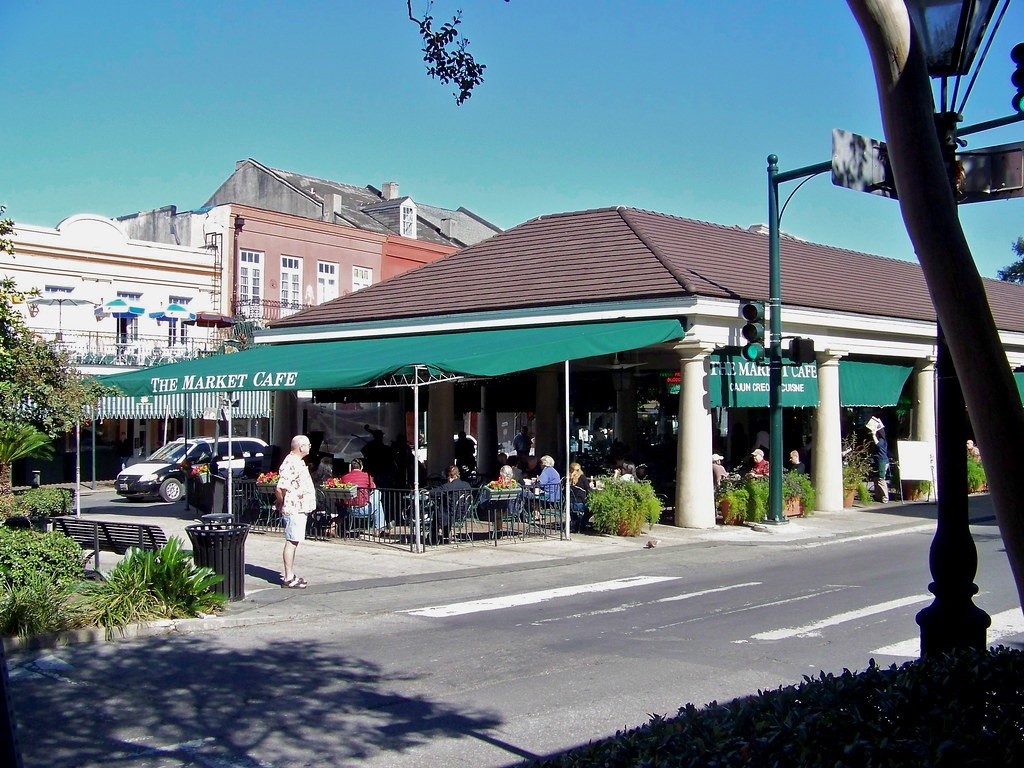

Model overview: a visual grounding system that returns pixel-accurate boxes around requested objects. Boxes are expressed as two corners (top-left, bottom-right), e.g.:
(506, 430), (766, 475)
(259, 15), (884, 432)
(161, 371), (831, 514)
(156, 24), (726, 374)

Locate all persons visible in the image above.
(563, 458), (591, 533)
(308, 454), (342, 539)
(360, 426), (414, 524)
(113, 430), (134, 476)
(274, 434), (316, 588)
(611, 451), (638, 474)
(718, 423), (756, 465)
(492, 452), (541, 517)
(333, 457), (396, 534)
(514, 426), (532, 458)
(872, 426), (890, 505)
(841, 441), (854, 467)
(712, 453), (730, 487)
(789, 449), (805, 474)
(751, 448), (770, 474)
(536, 454), (561, 510)
(966, 440), (982, 492)
(419, 466), (472, 543)
(454, 431), (476, 464)
(745, 424), (770, 463)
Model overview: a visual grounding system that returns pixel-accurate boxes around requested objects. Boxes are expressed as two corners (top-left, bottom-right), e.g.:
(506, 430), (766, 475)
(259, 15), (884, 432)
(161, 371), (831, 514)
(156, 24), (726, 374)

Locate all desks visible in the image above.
(107, 343), (137, 365)
(48, 341), (76, 357)
(155, 345), (188, 366)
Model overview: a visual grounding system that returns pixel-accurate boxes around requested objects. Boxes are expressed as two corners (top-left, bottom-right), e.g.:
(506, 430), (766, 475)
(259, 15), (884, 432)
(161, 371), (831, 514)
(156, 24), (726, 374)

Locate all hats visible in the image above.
(713, 453), (724, 461)
(751, 449), (764, 456)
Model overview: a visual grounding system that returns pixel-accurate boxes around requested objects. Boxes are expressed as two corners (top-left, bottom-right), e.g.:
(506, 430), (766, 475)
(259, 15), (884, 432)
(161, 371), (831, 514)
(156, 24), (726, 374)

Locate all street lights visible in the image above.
(914, 1), (1023, 665)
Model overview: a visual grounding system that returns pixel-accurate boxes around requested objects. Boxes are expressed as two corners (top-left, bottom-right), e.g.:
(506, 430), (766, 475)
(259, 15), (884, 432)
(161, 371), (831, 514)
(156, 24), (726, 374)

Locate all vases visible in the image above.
(197, 472), (208, 484)
(256, 483), (358, 500)
(482, 485), (523, 500)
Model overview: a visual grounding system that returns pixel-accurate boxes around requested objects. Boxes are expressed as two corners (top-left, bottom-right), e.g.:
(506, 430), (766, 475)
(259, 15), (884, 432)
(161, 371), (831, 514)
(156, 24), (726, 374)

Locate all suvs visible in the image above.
(113, 435), (270, 504)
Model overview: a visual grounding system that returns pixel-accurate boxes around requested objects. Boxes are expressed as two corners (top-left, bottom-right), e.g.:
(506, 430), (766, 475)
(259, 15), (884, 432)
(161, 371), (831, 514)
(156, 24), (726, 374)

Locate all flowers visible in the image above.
(487, 476), (519, 489)
(256, 471), (353, 489)
(191, 465), (210, 476)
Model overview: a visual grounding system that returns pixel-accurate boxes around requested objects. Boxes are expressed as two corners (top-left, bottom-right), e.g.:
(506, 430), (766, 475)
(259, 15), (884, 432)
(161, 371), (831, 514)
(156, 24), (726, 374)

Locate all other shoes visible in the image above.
(376, 531), (390, 537)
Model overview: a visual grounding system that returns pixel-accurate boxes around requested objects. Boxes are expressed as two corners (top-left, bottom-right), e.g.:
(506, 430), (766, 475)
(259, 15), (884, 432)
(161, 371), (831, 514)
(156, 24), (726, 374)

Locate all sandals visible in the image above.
(281, 575), (307, 588)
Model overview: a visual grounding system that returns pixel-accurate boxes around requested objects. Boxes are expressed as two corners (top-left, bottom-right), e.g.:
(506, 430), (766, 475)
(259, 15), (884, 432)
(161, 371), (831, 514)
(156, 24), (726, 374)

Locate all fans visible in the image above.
(592, 352), (647, 369)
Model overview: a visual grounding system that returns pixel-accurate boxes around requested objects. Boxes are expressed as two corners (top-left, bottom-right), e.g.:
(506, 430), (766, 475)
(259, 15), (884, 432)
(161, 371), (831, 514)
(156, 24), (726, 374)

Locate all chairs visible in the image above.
(256, 478), (567, 541)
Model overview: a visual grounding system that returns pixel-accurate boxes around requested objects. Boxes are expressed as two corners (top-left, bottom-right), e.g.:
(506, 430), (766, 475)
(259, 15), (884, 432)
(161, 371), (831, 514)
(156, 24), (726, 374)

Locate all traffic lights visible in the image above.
(739, 298), (765, 363)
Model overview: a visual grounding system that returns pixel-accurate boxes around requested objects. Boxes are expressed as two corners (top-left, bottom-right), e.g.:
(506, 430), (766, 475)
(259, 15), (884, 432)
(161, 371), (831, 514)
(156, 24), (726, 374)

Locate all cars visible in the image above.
(319, 433), (389, 463)
(453, 433), (477, 458)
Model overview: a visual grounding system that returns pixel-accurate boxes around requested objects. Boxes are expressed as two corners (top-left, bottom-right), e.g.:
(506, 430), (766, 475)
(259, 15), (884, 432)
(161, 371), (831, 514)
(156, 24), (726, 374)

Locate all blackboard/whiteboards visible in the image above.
(897, 441), (935, 479)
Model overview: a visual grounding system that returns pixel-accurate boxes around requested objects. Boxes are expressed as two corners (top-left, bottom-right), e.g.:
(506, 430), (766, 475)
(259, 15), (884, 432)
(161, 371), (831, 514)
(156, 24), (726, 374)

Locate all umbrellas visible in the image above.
(28, 291), (99, 330)
(95, 296), (147, 342)
(197, 310), (235, 346)
(150, 303), (196, 348)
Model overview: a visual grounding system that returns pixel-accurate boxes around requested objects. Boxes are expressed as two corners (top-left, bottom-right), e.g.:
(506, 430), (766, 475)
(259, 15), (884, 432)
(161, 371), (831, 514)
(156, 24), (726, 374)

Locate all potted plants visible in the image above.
(842, 463), (864, 508)
(894, 461), (931, 500)
(716, 485), (750, 525)
(967, 455), (986, 495)
(781, 468), (818, 517)
(585, 474), (663, 537)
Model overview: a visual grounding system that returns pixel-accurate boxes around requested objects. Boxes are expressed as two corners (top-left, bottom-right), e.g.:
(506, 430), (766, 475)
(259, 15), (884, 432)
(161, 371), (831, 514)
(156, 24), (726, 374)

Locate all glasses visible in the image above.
(304, 443), (312, 448)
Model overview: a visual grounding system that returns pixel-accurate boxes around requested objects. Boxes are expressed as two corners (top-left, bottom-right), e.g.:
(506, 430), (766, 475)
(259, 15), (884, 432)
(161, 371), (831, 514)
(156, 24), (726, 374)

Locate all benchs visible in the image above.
(53, 516), (194, 568)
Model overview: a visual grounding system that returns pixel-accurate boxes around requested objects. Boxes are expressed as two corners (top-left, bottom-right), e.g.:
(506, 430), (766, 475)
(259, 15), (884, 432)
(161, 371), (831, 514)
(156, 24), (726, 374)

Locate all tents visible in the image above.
(73, 316), (684, 555)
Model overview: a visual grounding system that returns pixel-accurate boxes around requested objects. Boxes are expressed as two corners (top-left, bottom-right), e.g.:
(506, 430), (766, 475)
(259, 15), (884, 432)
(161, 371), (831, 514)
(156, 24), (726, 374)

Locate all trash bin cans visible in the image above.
(30, 470), (42, 489)
(184, 522), (251, 601)
